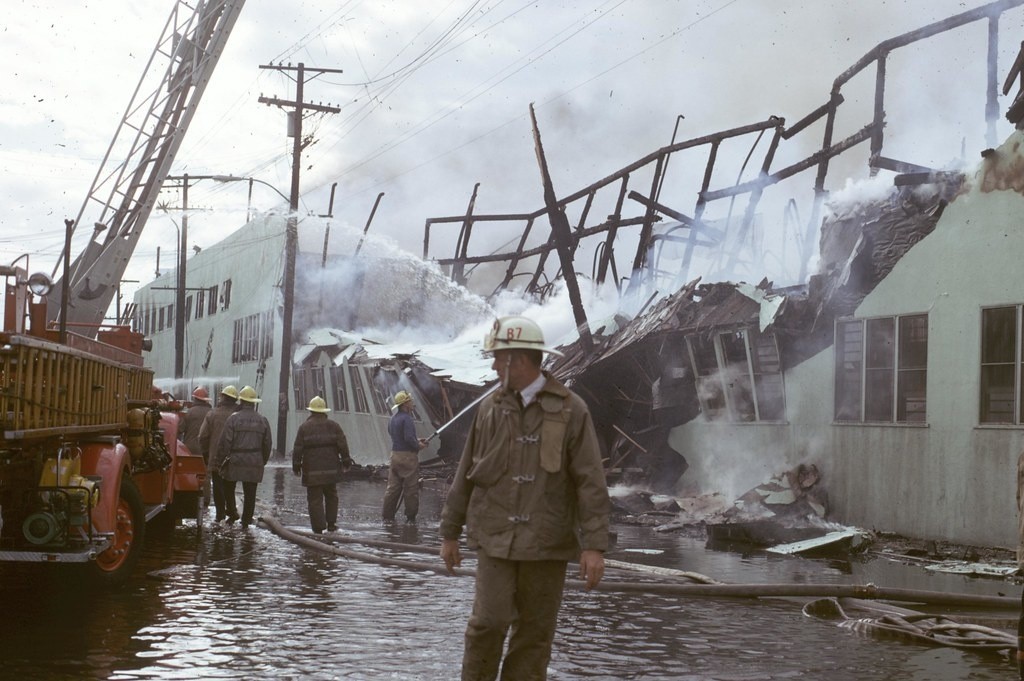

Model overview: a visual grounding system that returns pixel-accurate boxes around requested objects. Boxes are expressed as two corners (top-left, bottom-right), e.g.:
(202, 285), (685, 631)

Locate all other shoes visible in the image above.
(227, 513), (239, 525)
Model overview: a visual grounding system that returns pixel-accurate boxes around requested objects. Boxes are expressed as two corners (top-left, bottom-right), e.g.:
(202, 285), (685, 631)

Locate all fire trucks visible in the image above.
(1, 1), (247, 590)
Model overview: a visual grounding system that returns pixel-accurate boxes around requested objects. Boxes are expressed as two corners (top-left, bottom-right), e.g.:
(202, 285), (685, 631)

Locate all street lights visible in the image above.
(210, 173), (305, 466)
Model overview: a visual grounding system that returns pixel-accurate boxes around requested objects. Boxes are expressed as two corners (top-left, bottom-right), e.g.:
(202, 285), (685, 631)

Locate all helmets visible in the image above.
(483, 316), (565, 358)
(192, 388), (213, 401)
(222, 385), (238, 399)
(238, 386), (262, 403)
(306, 395), (331, 412)
(391, 389), (414, 411)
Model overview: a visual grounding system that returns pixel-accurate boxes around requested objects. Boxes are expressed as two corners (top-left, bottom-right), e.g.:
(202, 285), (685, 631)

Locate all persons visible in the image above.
(179, 384), (272, 527)
(293, 395), (353, 536)
(382, 390), (429, 527)
(439, 315), (612, 681)
